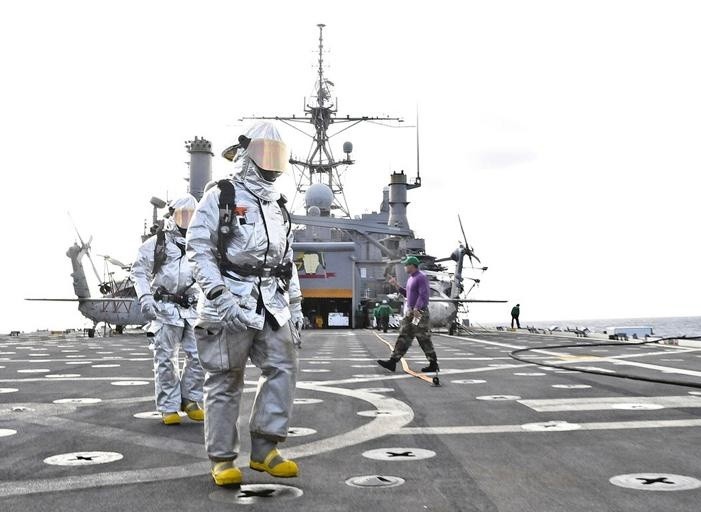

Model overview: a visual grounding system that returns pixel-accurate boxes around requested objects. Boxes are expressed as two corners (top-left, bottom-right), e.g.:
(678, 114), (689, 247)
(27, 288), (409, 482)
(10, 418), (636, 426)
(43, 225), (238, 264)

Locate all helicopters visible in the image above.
(24, 210), (155, 339)
(386, 214), (510, 329)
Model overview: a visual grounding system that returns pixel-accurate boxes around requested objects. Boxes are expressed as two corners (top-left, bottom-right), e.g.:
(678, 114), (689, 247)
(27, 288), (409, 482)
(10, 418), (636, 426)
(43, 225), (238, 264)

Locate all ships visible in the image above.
(0, 22), (701, 511)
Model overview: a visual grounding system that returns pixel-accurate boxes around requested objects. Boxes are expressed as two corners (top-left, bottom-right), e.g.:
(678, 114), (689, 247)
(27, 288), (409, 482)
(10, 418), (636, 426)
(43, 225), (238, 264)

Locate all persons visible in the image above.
(510, 304), (520, 329)
(379, 300), (395, 333)
(185, 124), (298, 486)
(355, 302), (366, 328)
(377, 256), (440, 373)
(133, 191), (204, 425)
(373, 303), (381, 330)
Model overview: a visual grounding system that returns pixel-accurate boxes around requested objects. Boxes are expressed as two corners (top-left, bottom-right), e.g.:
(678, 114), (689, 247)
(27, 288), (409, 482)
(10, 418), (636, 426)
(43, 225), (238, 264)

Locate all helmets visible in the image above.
(236, 121), (289, 185)
(169, 193), (198, 229)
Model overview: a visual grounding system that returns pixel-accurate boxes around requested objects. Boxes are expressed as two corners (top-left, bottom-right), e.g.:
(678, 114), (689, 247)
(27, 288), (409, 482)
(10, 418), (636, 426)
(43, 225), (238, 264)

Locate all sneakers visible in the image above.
(377, 357), (396, 371)
(375, 300), (388, 307)
(421, 361), (440, 372)
(249, 447), (299, 478)
(162, 411), (182, 425)
(211, 459), (243, 486)
(181, 398), (205, 421)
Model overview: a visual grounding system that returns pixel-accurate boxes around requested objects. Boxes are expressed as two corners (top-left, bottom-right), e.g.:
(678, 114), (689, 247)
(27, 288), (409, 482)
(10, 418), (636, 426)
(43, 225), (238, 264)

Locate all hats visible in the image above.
(401, 256), (420, 266)
